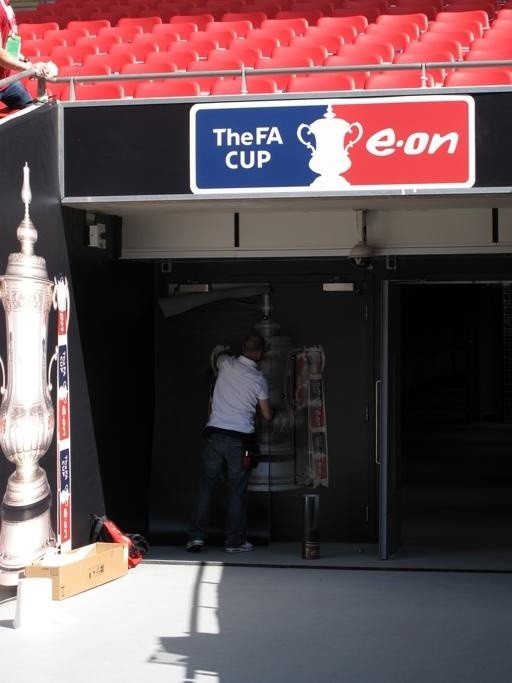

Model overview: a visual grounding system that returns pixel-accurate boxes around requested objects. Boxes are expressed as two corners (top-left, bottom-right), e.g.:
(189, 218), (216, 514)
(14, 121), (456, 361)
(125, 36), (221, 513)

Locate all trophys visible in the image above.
(296, 105), (364, 187)
(0, 161), (70, 588)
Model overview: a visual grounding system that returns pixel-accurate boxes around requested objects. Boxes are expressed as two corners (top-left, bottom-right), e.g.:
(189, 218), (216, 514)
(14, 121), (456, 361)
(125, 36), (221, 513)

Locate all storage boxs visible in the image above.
(21, 535), (133, 602)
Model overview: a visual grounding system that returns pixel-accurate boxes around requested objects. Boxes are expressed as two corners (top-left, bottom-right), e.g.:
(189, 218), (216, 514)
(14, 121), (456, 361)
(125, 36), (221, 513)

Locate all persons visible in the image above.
(186, 333), (273, 554)
(0, 0), (50, 111)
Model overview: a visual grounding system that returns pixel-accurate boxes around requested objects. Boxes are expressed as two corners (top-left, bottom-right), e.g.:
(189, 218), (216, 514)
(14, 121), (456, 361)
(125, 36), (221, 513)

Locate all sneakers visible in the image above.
(225, 540), (255, 554)
(183, 537), (204, 552)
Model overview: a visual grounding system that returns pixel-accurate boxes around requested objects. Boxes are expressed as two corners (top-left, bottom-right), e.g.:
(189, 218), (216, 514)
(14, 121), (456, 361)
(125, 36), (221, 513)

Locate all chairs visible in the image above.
(0, 0), (511, 125)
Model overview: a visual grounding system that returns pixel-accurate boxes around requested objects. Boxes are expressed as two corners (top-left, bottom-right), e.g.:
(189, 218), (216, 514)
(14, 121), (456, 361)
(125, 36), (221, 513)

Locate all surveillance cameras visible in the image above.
(347, 243), (376, 269)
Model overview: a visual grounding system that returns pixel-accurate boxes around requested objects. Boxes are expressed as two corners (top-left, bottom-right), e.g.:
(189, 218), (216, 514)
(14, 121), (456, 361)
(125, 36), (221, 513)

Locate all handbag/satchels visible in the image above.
(242, 443), (261, 471)
